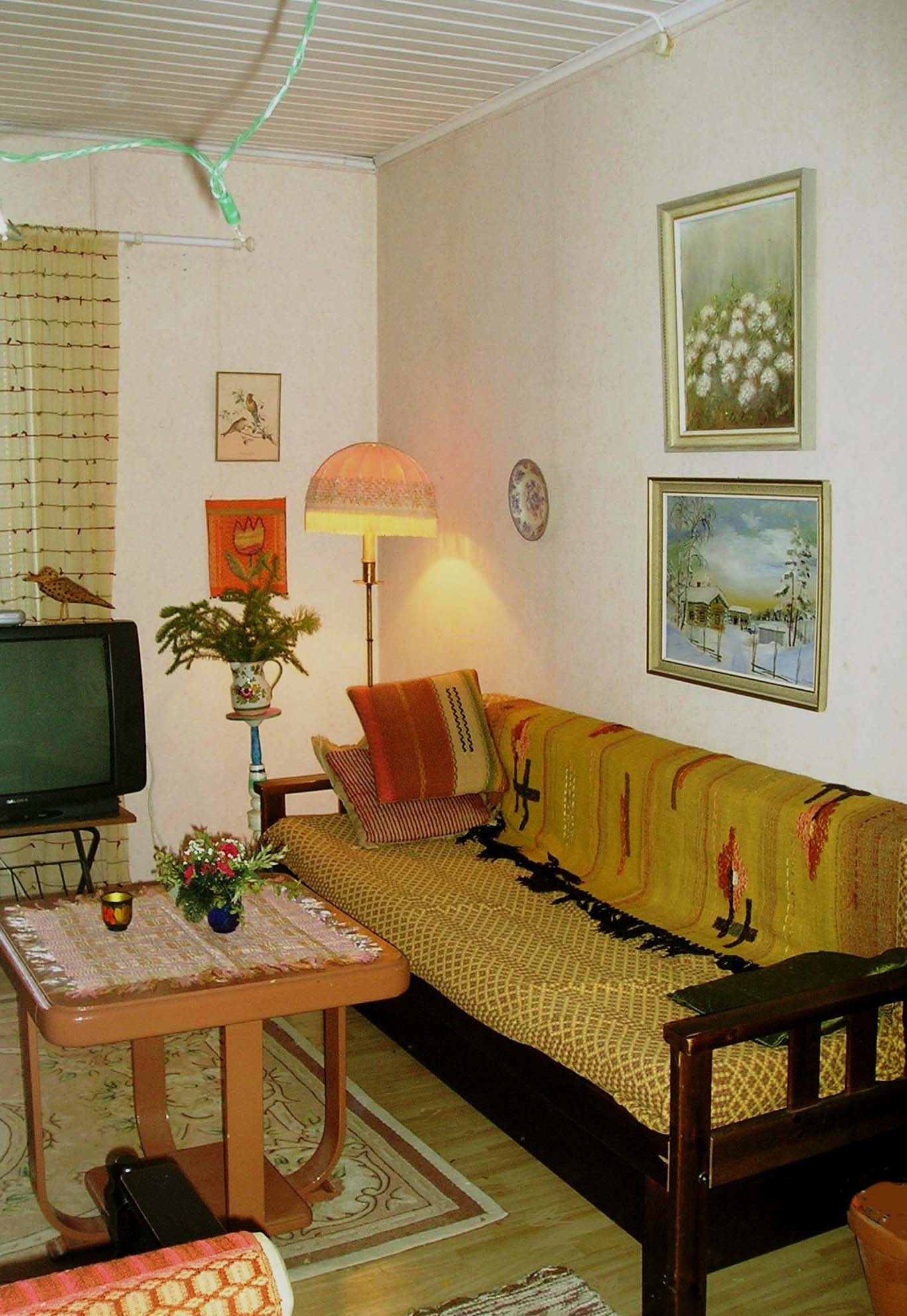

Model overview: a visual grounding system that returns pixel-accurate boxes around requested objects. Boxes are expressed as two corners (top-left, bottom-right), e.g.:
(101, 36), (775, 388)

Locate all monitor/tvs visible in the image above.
(1, 622), (146, 830)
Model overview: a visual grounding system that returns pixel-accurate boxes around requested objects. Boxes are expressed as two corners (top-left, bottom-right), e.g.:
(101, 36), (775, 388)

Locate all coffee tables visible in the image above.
(0, 872), (410, 1245)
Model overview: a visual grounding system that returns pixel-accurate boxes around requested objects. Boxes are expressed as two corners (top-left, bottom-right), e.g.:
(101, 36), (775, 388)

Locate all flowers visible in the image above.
(152, 829), (306, 930)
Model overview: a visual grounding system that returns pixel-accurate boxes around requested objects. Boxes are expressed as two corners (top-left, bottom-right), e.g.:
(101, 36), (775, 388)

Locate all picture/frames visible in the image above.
(646, 475), (832, 711)
(654, 167), (819, 456)
(215, 372), (282, 462)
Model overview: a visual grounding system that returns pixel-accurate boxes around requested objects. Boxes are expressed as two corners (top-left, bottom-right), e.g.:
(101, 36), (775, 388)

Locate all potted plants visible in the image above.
(154, 548), (322, 716)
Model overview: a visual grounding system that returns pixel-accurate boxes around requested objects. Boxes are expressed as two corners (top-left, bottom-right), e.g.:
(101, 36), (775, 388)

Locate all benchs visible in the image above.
(253, 693), (907, 1316)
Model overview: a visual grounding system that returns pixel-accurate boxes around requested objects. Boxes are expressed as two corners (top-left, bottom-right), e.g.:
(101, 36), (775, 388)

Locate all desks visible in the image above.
(0, 807), (137, 895)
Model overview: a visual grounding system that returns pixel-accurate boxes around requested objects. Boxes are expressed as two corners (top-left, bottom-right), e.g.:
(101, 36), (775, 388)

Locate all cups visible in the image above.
(100, 890), (133, 931)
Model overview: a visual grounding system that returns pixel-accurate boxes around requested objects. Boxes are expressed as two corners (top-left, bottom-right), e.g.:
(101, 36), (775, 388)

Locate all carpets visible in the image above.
(407, 1266), (617, 1316)
(0, 994), (509, 1285)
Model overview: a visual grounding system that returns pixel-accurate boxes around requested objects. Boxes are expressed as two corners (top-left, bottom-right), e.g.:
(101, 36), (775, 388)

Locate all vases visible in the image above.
(207, 889), (241, 935)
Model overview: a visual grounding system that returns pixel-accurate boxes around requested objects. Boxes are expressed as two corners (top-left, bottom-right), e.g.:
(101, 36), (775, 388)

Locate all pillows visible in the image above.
(346, 669), (510, 804)
(310, 734), (499, 849)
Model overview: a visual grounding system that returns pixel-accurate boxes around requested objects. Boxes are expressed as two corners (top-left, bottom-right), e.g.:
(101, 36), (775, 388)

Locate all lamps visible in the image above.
(304, 441), (440, 687)
